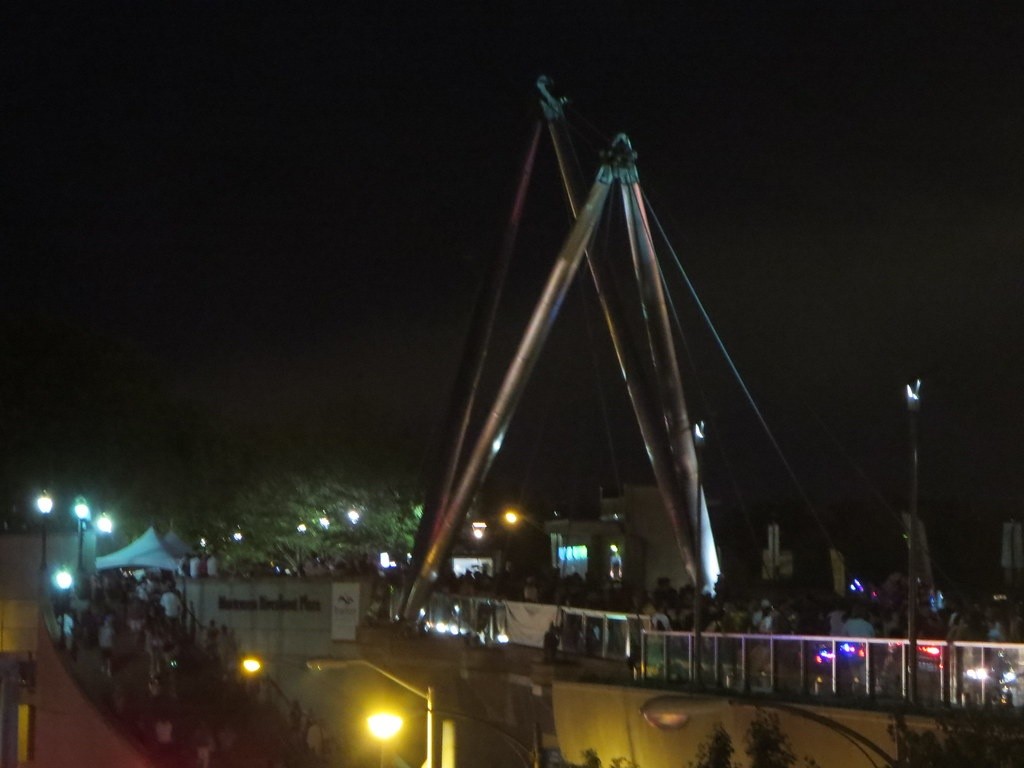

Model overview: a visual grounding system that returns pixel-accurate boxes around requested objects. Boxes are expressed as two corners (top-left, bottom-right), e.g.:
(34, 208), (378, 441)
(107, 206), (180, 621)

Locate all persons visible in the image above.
(0, 493), (31, 533)
(50, 566), (359, 768)
(376, 540), (1023, 716)
(177, 539), (374, 579)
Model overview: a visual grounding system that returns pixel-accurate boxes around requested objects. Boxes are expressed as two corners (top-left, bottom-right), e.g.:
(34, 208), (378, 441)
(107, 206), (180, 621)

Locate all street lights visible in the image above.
(71, 498), (91, 619)
(35, 493), (54, 616)
(318, 515), (332, 608)
(200, 539), (210, 585)
(94, 511), (112, 624)
(506, 511), (562, 586)
(232, 529), (248, 592)
(347, 507), (371, 611)
(470, 521), (498, 647)
(691, 422), (704, 687)
(56, 569), (74, 645)
(639, 692), (900, 768)
(302, 655), (435, 767)
(294, 524), (307, 607)
(901, 380), (918, 704)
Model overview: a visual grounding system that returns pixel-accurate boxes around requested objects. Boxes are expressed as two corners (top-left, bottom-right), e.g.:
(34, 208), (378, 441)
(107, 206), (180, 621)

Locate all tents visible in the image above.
(94, 524), (200, 577)
